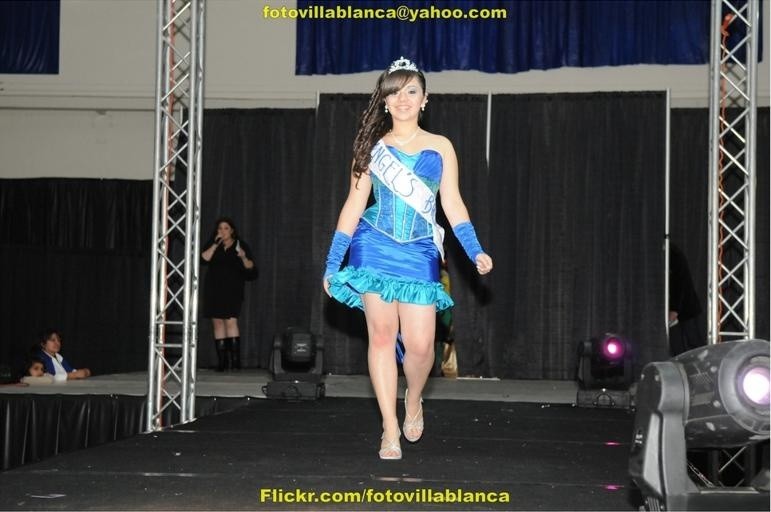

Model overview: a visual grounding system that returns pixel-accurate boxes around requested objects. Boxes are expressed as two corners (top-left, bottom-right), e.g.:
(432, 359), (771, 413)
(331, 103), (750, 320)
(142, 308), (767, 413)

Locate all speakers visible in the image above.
(214, 337), (229, 373)
(226, 335), (241, 374)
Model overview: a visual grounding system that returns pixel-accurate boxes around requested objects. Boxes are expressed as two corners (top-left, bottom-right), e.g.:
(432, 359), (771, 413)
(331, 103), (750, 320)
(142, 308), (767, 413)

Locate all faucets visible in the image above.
(378, 420), (403, 461)
(403, 388), (424, 444)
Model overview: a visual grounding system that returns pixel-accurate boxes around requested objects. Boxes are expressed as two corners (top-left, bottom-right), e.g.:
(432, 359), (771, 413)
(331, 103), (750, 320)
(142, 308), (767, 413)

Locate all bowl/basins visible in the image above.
(389, 127), (419, 148)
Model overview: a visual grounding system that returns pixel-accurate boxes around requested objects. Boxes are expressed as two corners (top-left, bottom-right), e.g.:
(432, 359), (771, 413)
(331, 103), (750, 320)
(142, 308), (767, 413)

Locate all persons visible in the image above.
(17, 356), (54, 384)
(323, 56), (493, 460)
(37, 330), (90, 382)
(668, 244), (702, 356)
(438, 255), (458, 378)
(201, 217), (259, 372)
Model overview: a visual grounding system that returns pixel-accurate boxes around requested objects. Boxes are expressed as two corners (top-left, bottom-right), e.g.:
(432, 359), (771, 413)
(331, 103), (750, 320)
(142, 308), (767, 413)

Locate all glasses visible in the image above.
(631, 338), (770, 510)
(571, 330), (637, 410)
(261, 321), (330, 403)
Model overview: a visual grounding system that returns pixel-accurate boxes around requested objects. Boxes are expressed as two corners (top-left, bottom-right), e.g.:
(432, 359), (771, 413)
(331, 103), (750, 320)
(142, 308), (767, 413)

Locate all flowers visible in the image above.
(215, 238), (221, 243)
